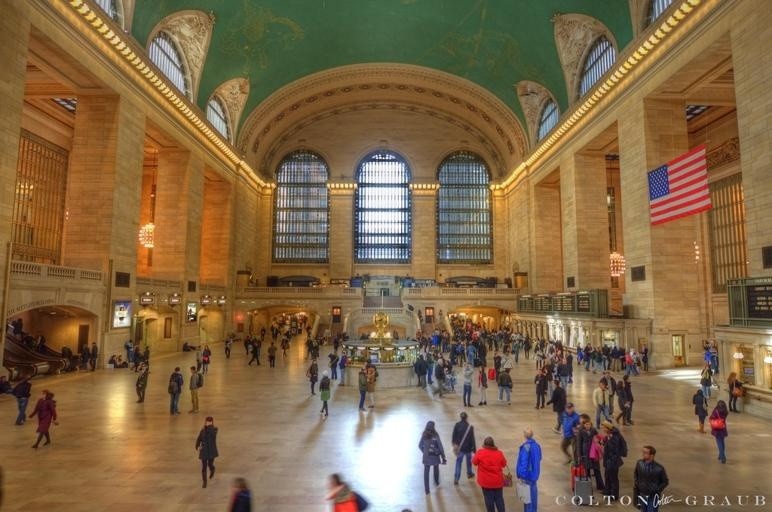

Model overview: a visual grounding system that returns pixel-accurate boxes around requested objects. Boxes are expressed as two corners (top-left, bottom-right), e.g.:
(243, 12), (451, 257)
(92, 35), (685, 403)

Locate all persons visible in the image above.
(0, 303), (747, 512)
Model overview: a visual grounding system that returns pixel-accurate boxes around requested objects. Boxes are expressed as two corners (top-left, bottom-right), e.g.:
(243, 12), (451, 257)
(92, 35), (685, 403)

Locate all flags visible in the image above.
(646, 143), (713, 227)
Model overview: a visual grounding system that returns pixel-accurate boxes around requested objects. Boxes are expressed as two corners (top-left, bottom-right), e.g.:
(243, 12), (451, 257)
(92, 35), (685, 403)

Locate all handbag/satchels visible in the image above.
(702, 402), (707, 410)
(711, 418), (724, 429)
(351, 493), (367, 511)
(732, 388), (741, 397)
(504, 473), (512, 486)
(516, 482), (531, 504)
(452, 444), (460, 455)
(428, 440), (439, 456)
(168, 382), (178, 391)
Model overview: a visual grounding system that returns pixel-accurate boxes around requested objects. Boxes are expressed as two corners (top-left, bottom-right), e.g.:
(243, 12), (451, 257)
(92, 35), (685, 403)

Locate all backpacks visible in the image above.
(196, 374), (203, 387)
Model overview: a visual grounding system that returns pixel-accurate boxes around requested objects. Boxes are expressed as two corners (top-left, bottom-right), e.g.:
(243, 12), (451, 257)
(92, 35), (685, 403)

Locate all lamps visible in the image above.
(608, 157), (627, 278)
(137, 141), (160, 249)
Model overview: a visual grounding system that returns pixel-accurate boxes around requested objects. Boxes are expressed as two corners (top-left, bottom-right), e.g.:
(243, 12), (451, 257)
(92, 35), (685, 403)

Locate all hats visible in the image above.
(602, 418), (613, 429)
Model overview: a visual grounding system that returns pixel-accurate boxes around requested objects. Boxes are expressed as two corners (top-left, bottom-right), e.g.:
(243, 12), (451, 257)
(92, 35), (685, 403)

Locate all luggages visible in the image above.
(572, 456), (593, 505)
